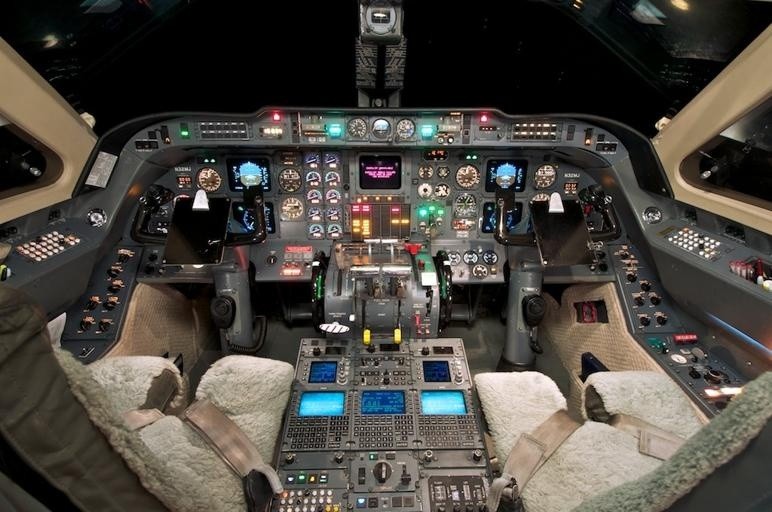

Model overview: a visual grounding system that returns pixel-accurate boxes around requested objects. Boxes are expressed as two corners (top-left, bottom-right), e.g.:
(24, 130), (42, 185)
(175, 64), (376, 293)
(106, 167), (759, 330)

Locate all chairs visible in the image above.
(1, 277), (296, 511)
(471, 370), (772, 512)
